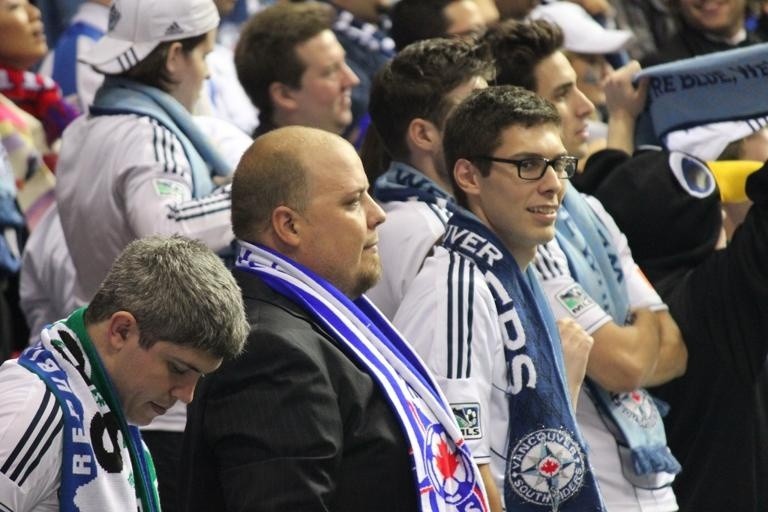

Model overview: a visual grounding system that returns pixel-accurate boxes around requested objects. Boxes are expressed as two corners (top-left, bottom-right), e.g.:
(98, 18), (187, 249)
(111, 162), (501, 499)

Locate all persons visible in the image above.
(190, 122), (491, 511)
(53, 0), (256, 511)
(589, 147), (767, 511)
(473, 14), (692, 512)
(1, 1), (767, 368)
(385, 81), (608, 512)
(0, 231), (253, 512)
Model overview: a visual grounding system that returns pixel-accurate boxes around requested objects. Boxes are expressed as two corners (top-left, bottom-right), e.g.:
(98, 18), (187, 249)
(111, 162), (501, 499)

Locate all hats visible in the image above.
(525, 2), (633, 54)
(79, 1), (219, 75)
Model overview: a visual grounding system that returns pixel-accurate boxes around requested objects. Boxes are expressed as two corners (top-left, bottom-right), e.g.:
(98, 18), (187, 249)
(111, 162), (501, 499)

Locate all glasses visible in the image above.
(472, 153), (579, 181)
